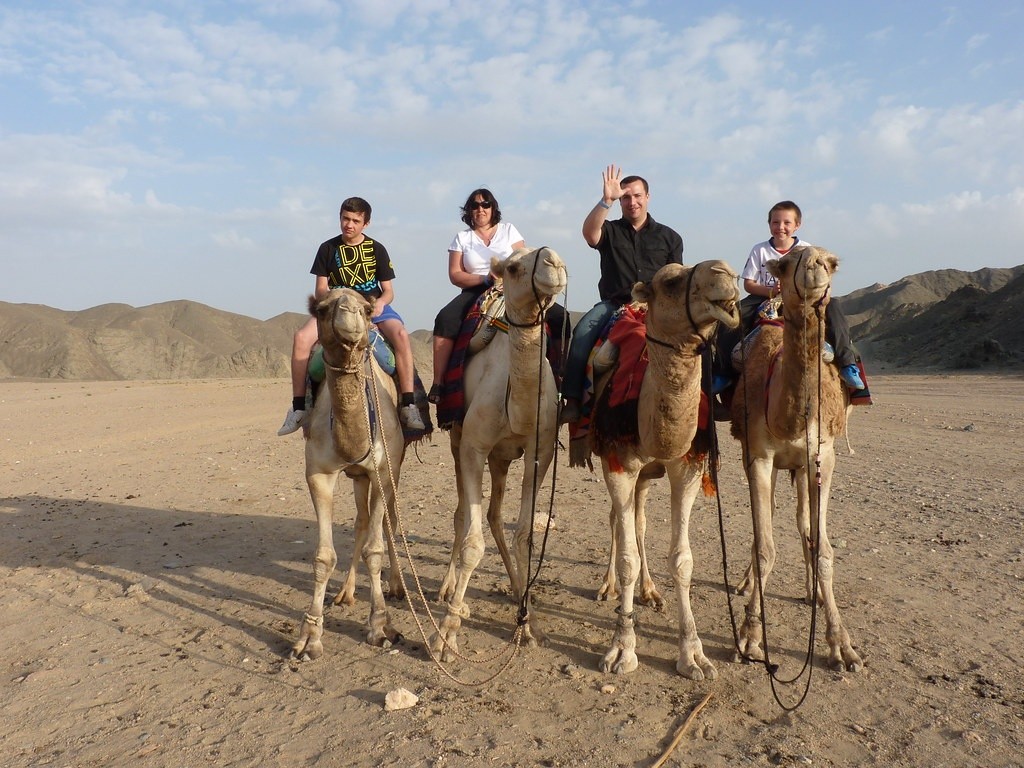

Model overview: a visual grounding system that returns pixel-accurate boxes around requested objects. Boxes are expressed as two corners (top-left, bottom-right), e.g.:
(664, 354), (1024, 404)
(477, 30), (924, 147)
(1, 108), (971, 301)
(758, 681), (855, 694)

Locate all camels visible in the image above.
(287, 284), (435, 662)
(427, 245), (573, 663)
(721, 245), (871, 673)
(570, 259), (740, 682)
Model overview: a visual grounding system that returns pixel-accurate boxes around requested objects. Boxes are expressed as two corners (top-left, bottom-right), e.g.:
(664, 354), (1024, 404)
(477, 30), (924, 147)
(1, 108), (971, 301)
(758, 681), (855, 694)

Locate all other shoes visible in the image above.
(562, 400), (577, 423)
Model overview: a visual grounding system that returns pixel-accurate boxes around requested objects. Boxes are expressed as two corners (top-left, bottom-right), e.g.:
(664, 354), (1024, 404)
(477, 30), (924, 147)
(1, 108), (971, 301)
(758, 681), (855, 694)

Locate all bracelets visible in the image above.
(485, 275), (490, 285)
(599, 199), (613, 209)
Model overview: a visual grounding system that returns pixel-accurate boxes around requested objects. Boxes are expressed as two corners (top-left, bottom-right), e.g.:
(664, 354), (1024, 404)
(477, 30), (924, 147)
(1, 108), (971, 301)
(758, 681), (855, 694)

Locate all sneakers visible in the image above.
(400, 404), (425, 429)
(712, 377), (731, 393)
(839, 364), (864, 389)
(278, 407), (306, 436)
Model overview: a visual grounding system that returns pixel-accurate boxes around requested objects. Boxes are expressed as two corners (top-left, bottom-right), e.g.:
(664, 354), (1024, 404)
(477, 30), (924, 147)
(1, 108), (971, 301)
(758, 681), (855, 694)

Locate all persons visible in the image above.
(428, 188), (572, 405)
(558, 163), (712, 424)
(277, 196), (426, 436)
(711, 200), (868, 396)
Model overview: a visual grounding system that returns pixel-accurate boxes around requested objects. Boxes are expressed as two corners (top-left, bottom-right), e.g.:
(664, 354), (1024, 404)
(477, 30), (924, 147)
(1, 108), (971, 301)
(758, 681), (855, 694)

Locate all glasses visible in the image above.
(469, 201), (491, 210)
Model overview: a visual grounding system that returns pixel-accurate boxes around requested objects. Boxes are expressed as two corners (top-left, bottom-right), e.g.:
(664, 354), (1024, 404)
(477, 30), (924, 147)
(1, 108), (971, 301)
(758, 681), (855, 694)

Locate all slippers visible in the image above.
(427, 383), (443, 404)
